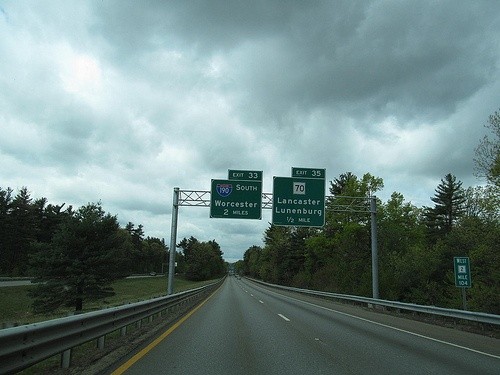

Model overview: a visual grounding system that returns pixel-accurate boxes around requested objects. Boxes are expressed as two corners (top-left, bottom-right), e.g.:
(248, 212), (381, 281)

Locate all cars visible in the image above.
(229, 272), (241, 280)
(149, 272), (157, 276)
